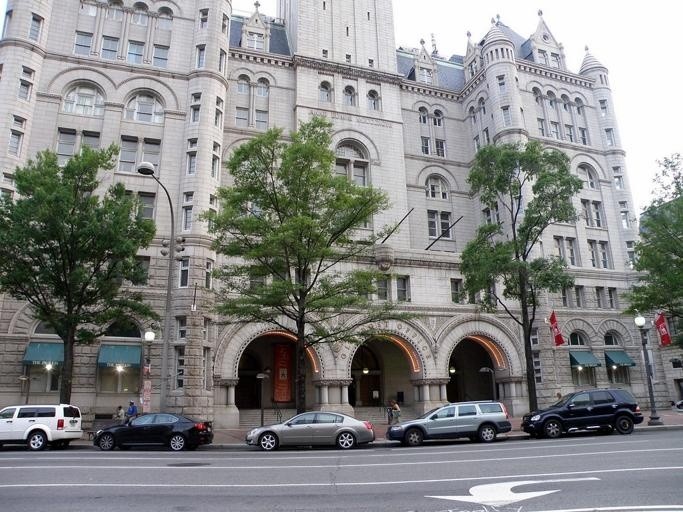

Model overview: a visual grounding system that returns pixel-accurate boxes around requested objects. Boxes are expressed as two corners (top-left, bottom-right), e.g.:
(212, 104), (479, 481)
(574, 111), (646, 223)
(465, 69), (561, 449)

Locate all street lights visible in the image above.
(633, 312), (665, 425)
(137, 159), (176, 415)
(143, 331), (155, 381)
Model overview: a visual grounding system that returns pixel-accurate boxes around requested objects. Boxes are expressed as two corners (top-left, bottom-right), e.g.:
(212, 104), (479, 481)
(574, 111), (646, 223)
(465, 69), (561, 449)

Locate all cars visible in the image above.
(91, 412), (214, 452)
(244, 410), (376, 450)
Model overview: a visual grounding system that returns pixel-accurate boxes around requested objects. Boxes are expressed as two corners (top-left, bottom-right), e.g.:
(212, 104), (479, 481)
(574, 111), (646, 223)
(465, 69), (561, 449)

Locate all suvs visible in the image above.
(386, 398), (512, 447)
(520, 386), (645, 439)
(0, 403), (83, 452)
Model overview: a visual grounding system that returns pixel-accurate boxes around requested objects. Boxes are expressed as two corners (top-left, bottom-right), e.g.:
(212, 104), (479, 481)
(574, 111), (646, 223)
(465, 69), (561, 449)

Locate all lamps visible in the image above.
(449, 365), (456, 374)
(362, 365), (370, 375)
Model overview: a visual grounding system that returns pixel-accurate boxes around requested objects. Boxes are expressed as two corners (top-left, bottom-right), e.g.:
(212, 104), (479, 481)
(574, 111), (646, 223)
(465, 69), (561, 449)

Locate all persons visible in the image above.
(117, 405), (124, 425)
(557, 392), (562, 400)
(387, 407), (393, 425)
(125, 400), (137, 423)
(391, 399), (401, 425)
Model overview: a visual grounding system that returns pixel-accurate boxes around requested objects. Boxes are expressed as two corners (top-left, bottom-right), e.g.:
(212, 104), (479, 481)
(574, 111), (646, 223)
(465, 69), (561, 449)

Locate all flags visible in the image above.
(549, 307), (566, 346)
(654, 311), (672, 345)
